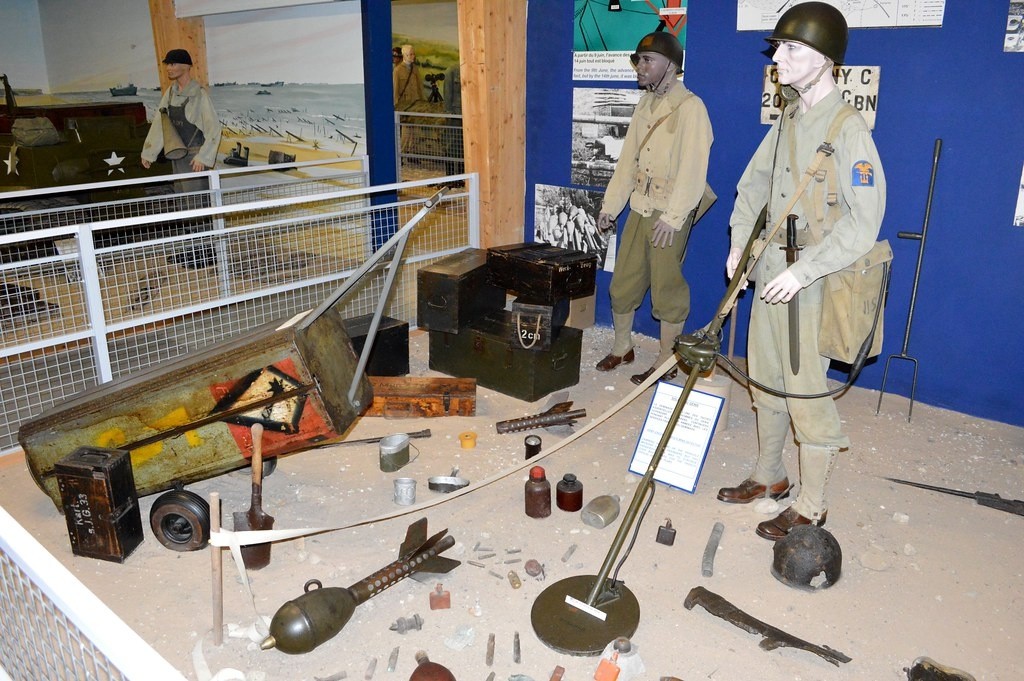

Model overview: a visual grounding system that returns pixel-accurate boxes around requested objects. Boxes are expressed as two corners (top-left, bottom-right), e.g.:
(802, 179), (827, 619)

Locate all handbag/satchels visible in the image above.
(693, 182), (717, 224)
(817, 238), (895, 364)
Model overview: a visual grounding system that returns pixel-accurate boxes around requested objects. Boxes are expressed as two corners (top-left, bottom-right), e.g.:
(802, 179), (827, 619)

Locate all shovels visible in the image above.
(233, 423), (274, 570)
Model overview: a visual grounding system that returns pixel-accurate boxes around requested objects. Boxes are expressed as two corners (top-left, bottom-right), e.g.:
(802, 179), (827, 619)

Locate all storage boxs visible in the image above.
(417, 241), (598, 402)
(54, 446), (145, 561)
(343, 313), (412, 377)
(356, 376), (479, 416)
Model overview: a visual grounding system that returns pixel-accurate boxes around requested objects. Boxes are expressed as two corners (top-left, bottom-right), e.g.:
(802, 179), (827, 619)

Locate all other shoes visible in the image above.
(168, 250), (218, 269)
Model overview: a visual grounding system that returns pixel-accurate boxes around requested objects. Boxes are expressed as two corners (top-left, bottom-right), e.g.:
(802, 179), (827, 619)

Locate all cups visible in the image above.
(393, 478), (417, 506)
(525, 435), (542, 460)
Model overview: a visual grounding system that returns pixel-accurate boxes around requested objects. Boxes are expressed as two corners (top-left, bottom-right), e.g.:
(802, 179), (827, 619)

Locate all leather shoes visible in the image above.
(597, 346), (634, 372)
(756, 505), (829, 540)
(718, 477), (790, 503)
(632, 367), (679, 386)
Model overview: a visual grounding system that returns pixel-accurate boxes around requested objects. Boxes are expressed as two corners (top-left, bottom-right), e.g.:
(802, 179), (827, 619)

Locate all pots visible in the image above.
(427, 468), (470, 494)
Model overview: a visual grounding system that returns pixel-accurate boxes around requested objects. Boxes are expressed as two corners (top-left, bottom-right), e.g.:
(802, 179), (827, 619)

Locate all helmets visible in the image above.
(630, 32), (685, 74)
(764, 2), (849, 66)
(163, 49), (193, 65)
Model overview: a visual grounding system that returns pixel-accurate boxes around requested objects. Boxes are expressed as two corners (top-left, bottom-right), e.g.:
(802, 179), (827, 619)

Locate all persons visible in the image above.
(393, 44), (423, 166)
(594, 33), (714, 386)
(139, 48), (222, 269)
(717, 2), (885, 540)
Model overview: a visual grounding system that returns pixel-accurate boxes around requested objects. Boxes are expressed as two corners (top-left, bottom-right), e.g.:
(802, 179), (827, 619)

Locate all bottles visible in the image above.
(581, 495), (620, 530)
(525, 466), (552, 519)
(556, 474), (583, 513)
